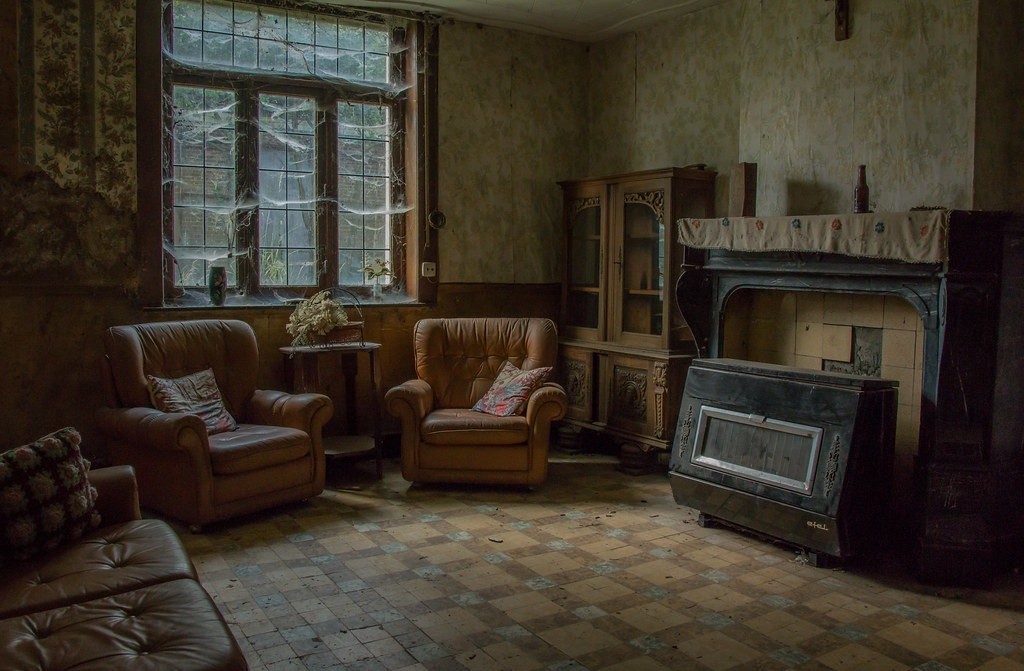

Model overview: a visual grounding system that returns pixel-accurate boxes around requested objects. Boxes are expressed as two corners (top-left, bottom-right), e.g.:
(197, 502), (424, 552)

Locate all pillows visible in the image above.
(1, 427), (102, 567)
(470, 360), (553, 417)
(146, 367), (240, 436)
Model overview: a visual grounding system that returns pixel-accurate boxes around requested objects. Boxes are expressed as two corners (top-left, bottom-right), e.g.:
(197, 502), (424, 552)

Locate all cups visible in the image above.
(209, 266), (228, 307)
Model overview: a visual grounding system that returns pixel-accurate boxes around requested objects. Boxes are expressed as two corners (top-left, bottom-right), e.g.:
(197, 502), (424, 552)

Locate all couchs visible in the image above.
(387, 316), (567, 491)
(96, 317), (335, 533)
(0, 464), (250, 671)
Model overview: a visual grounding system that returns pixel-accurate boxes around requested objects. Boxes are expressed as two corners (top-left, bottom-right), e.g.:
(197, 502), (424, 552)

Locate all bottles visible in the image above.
(853, 164), (869, 213)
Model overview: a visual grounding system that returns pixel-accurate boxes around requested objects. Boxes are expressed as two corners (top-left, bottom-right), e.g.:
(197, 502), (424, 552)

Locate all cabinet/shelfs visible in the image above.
(551, 166), (720, 353)
(554, 342), (695, 450)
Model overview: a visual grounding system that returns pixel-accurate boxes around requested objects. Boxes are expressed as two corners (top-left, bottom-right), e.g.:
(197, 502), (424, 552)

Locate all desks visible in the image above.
(279, 343), (384, 484)
